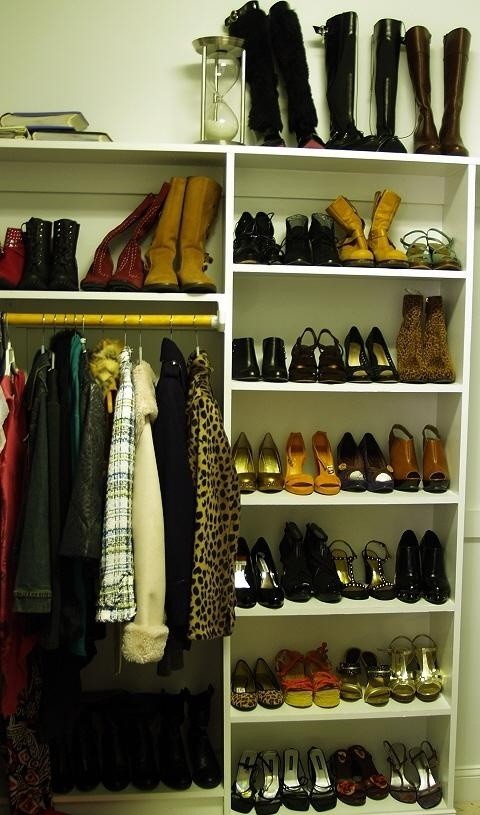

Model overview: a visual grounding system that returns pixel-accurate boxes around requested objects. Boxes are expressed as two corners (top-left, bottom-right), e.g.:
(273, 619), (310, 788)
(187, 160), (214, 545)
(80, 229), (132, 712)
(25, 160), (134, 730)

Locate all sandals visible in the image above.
(231, 740), (442, 815)
(233, 424), (450, 495)
(231, 634), (442, 710)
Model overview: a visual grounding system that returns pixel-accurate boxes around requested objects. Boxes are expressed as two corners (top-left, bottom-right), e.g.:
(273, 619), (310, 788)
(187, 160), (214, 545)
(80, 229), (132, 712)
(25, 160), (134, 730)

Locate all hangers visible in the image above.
(0, 312), (201, 376)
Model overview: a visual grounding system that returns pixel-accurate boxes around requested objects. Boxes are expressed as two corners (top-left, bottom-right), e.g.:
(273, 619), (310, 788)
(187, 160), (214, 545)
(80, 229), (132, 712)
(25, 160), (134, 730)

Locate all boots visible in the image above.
(233, 189), (461, 269)
(48, 685), (221, 793)
(225, 0), (471, 155)
(0, 176), (222, 292)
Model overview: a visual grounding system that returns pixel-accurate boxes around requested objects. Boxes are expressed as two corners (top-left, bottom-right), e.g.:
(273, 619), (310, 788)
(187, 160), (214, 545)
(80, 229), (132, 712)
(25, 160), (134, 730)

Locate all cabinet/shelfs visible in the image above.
(0, 137), (477, 815)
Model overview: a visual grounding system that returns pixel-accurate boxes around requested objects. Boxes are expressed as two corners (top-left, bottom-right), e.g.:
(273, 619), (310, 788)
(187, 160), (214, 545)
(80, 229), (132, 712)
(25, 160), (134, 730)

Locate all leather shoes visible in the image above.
(234, 521), (450, 608)
(232, 295), (455, 383)
